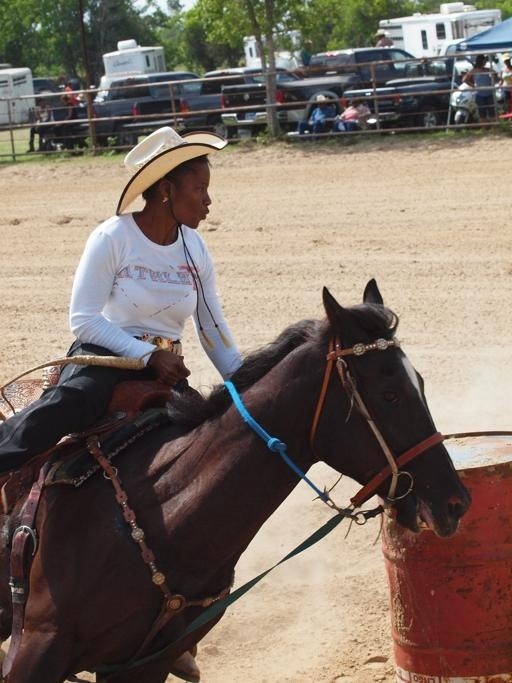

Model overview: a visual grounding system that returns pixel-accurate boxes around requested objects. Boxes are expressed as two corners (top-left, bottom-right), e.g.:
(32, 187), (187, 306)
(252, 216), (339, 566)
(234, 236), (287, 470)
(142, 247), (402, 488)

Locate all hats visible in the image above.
(316, 94), (327, 101)
(114, 121), (229, 219)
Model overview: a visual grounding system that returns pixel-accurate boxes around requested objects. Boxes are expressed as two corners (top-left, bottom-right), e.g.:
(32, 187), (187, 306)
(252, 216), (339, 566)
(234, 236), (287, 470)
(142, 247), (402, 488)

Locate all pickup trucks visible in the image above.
(35, 16), (512, 156)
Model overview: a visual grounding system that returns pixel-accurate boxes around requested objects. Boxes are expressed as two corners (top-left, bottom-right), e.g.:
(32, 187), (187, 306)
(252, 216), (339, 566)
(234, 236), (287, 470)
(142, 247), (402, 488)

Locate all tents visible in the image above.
(445, 15), (512, 132)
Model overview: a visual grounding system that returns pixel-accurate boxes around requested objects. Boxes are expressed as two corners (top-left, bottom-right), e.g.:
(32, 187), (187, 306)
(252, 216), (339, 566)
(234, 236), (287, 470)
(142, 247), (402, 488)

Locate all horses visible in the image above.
(0, 278), (474, 683)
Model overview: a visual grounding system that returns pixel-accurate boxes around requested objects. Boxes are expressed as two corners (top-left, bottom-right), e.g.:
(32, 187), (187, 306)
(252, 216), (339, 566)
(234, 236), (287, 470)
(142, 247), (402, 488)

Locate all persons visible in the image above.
(296, 92), (372, 140)
(373, 30), (394, 48)
(300, 39), (313, 66)
(2, 122), (246, 683)
(449, 53), (511, 130)
(25, 85), (92, 151)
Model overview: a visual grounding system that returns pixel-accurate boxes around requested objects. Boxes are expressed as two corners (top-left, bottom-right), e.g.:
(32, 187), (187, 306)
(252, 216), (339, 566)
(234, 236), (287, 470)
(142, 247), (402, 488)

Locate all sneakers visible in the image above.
(170, 652), (199, 683)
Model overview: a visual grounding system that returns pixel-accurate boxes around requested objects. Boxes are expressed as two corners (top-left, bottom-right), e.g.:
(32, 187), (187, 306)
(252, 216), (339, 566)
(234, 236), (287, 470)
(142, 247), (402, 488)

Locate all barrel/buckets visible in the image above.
(380, 432), (512, 683)
